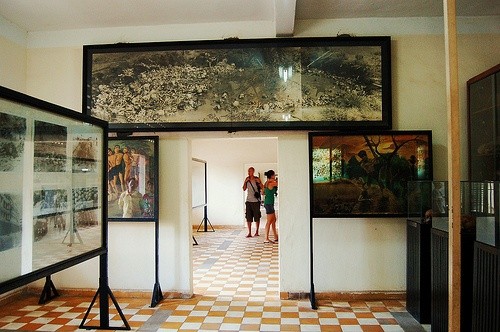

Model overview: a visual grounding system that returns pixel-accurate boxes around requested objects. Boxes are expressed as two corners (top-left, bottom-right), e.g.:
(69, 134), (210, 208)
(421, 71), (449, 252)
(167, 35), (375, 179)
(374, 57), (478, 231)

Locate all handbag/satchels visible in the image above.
(254, 192), (261, 200)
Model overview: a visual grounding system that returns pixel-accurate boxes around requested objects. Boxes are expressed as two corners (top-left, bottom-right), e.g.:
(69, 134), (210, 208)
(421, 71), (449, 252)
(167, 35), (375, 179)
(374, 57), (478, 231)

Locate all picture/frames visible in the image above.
(82, 36), (392, 132)
(307, 130), (433, 218)
(0, 85), (108, 295)
(107, 136), (160, 222)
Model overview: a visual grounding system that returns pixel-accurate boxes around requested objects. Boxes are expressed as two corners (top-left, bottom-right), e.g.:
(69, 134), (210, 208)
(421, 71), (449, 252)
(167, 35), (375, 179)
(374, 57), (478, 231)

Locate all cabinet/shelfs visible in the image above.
(466, 64), (500, 183)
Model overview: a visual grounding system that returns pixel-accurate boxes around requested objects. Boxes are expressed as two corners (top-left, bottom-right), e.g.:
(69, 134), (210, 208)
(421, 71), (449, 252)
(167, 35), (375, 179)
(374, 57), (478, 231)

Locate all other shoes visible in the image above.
(247, 235), (252, 237)
(254, 234), (259, 236)
(264, 240), (275, 243)
(274, 239), (278, 241)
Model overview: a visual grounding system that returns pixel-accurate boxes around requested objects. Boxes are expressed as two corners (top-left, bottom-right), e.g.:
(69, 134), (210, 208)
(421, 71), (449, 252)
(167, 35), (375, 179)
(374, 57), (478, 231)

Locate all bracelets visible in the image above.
(257, 182), (261, 184)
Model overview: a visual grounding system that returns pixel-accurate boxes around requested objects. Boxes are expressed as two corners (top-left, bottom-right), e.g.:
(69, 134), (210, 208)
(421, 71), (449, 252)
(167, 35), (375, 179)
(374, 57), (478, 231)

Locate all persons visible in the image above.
(119, 178), (144, 218)
(261, 170), (278, 243)
(243, 167), (264, 237)
(432, 181), (446, 213)
(141, 180), (154, 218)
